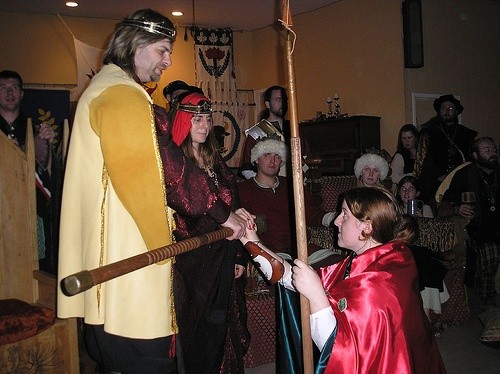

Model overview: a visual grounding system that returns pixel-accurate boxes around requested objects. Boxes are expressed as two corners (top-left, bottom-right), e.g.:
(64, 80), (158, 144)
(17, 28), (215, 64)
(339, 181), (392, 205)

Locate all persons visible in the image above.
(242, 86), (310, 179)
(394, 176), (433, 219)
(0, 70), (54, 161)
(354, 153), (388, 187)
(236, 139), (344, 267)
(163, 81), (188, 104)
(438, 136), (500, 341)
(58, 8), (179, 374)
(246, 187), (447, 374)
(417, 95), (479, 180)
(389, 124), (419, 186)
(159, 92), (256, 374)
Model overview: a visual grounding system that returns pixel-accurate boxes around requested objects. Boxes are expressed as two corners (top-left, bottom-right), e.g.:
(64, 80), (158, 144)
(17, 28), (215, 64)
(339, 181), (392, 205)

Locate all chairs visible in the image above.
(0, 118), (82, 374)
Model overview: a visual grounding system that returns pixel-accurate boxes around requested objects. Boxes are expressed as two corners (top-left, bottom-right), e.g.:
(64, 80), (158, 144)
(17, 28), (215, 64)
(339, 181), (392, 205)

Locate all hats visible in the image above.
(251, 139), (287, 167)
(434, 95), (463, 115)
(354, 153), (388, 180)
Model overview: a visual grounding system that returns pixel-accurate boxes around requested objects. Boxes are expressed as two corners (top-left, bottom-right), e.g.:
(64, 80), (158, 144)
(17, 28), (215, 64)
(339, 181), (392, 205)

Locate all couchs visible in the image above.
(245, 176), (470, 369)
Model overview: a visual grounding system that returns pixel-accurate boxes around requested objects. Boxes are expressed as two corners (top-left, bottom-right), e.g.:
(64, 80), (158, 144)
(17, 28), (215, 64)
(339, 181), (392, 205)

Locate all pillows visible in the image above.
(478, 308), (500, 344)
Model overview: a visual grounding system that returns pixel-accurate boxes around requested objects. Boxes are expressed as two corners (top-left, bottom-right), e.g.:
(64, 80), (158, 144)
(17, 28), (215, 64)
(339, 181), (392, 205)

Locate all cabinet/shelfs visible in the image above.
(298, 115), (382, 176)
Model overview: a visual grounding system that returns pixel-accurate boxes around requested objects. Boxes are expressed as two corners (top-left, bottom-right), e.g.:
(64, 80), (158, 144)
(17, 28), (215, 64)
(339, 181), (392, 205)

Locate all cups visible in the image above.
(461, 191), (475, 207)
(408, 198), (417, 217)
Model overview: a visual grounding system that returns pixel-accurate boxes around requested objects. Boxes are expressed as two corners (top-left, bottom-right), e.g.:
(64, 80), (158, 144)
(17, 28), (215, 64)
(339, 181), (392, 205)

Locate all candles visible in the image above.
(327, 97), (332, 102)
(335, 93), (339, 98)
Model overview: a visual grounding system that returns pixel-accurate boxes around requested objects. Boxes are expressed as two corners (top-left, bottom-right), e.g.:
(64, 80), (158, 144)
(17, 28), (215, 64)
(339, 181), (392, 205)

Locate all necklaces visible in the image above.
(439, 123), (457, 145)
